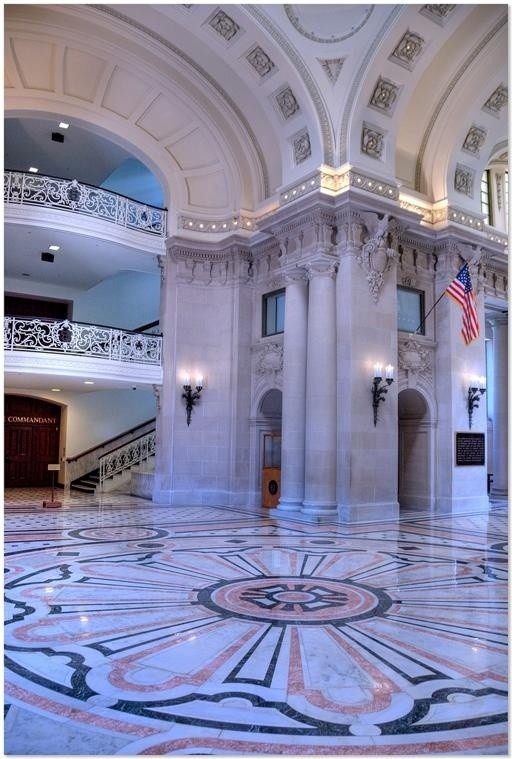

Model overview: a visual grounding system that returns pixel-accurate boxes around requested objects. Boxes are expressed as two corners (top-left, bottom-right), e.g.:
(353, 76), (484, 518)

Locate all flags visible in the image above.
(445, 263), (479, 343)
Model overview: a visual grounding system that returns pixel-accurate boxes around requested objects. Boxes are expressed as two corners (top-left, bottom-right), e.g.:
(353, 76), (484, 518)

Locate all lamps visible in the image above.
(370, 363), (394, 426)
(466, 377), (485, 428)
(179, 374), (204, 427)
